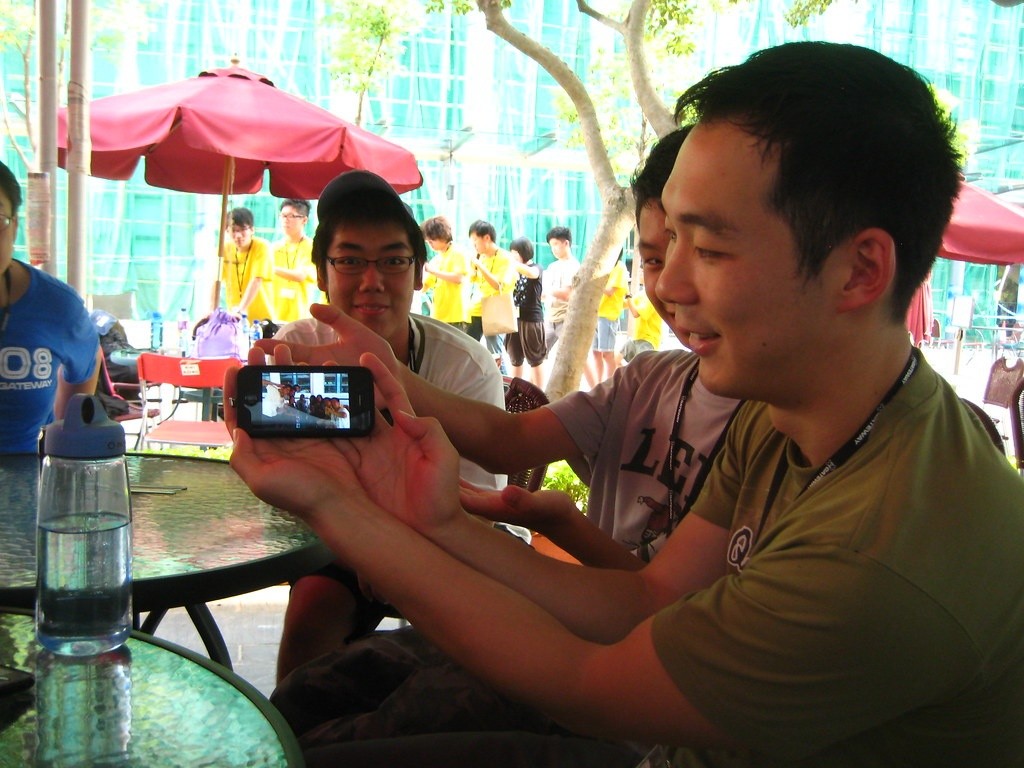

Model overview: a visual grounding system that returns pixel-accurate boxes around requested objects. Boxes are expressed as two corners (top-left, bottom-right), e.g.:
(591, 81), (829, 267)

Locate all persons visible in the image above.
(223, 42), (1024, 768)
(255, 124), (738, 748)
(216, 198), (660, 394)
(0, 161), (102, 455)
(263, 382), (348, 428)
(265, 170), (506, 682)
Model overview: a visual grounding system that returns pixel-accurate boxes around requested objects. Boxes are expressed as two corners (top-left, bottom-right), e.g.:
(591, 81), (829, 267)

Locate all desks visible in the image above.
(0, 451), (333, 669)
(0, 610), (305, 768)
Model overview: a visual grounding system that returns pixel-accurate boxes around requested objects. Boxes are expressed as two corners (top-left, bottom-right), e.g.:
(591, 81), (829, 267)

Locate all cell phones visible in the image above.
(236, 365), (374, 437)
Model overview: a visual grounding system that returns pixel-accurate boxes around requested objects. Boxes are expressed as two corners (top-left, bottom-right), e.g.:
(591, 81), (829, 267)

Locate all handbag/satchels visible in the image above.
(481, 283), (519, 337)
(195, 308), (242, 359)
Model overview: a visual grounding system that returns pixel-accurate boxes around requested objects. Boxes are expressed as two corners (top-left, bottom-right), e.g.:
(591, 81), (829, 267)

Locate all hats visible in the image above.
(317, 169), (428, 266)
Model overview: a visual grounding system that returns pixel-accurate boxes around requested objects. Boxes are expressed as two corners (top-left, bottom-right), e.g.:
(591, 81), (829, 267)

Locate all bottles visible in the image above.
(32, 392), (135, 662)
(237, 314), (251, 360)
(177, 308), (191, 348)
(250, 320), (264, 349)
(150, 311), (163, 351)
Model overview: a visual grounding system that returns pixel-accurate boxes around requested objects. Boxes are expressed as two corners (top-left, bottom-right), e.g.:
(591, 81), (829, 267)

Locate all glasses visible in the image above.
(0, 214), (15, 232)
(279, 213), (305, 222)
(322, 253), (421, 276)
(227, 226), (250, 236)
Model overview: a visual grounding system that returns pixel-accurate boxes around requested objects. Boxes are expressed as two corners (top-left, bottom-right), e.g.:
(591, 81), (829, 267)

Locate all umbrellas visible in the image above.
(55, 60), (424, 323)
(900, 172), (1023, 348)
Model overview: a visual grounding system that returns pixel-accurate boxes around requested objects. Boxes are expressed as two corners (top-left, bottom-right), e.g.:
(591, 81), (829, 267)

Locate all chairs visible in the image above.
(137, 354), (243, 461)
(100, 346), (162, 449)
